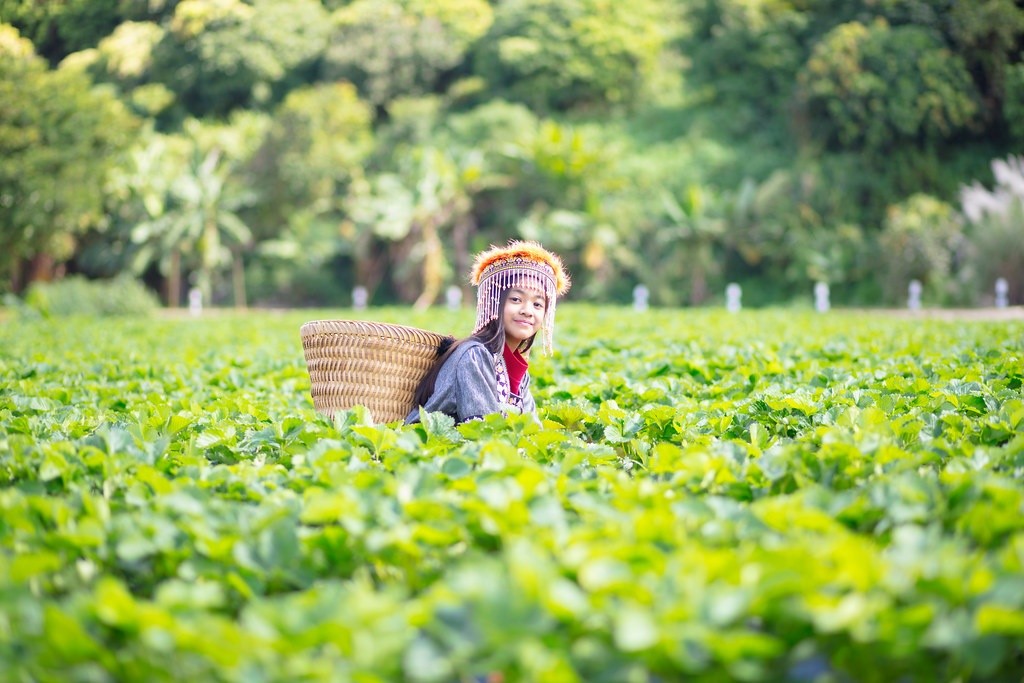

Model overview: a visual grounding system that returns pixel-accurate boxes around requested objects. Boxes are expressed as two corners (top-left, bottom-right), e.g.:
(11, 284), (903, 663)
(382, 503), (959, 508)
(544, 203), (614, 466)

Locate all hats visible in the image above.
(470, 241), (570, 356)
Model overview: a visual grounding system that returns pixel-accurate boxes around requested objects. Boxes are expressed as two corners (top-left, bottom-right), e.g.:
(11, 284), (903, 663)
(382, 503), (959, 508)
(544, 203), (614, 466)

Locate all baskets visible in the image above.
(300, 319), (458, 425)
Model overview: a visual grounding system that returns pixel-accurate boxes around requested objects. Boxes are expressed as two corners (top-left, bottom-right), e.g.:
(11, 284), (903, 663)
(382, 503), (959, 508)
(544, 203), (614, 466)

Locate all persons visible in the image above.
(395, 238), (571, 443)
